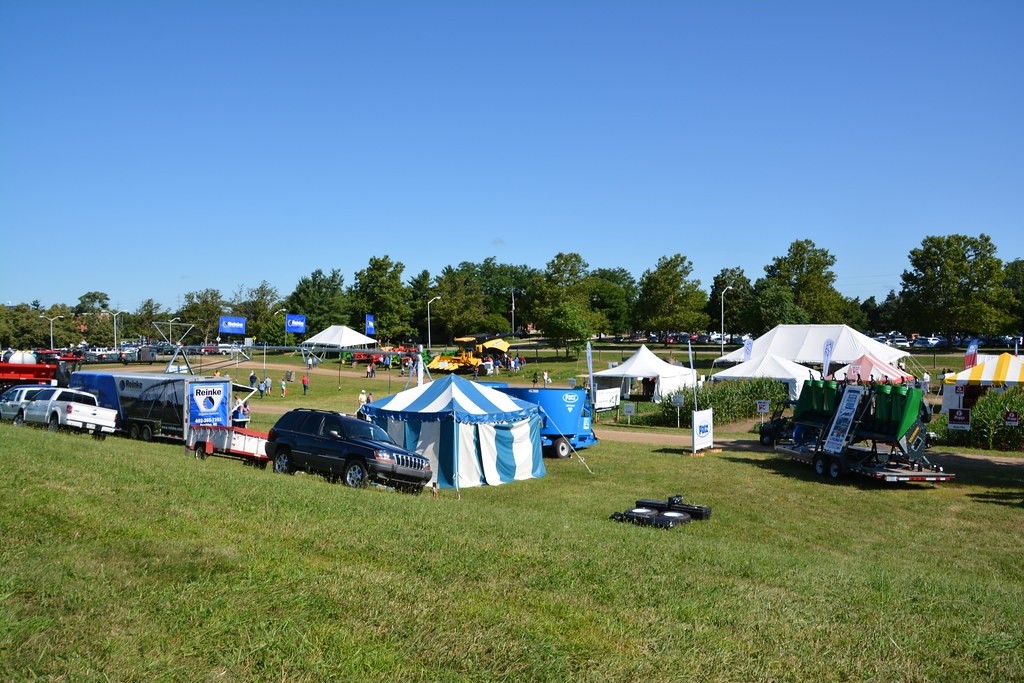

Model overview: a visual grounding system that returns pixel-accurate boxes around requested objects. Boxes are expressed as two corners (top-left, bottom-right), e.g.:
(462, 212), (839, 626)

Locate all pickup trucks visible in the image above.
(0, 387), (118, 442)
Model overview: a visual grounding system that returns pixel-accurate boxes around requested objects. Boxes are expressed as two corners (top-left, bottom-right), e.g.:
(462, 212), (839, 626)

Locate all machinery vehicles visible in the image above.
(427, 332), (510, 371)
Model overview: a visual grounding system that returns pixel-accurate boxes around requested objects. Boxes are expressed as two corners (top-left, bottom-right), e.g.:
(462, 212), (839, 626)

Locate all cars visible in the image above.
(203, 344), (216, 353)
(871, 333), (971, 348)
(678, 334), (690, 344)
(714, 337), (727, 344)
(662, 336), (674, 343)
(696, 335), (708, 345)
(977, 337), (1023, 345)
(613, 335), (624, 343)
(629, 334), (639, 342)
(647, 335), (658, 343)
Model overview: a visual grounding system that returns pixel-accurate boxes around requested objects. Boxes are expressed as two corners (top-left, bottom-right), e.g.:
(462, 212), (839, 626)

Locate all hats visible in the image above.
(361, 389), (366, 393)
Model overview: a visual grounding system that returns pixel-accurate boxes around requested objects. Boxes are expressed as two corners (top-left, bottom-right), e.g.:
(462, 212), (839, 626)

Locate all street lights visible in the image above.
(721, 286), (732, 356)
(168, 318), (180, 346)
(39, 316), (64, 350)
(428, 296), (441, 349)
(101, 309), (127, 350)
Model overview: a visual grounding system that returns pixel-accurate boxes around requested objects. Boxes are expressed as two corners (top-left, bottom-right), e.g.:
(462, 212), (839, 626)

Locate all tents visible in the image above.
(300, 325), (379, 364)
(939, 351), (1024, 416)
(710, 324), (932, 412)
(589, 343), (697, 404)
(356, 372), (548, 490)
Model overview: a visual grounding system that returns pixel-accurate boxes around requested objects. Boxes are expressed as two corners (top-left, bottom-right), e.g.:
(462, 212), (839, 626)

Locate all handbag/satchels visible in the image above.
(548, 378), (552, 383)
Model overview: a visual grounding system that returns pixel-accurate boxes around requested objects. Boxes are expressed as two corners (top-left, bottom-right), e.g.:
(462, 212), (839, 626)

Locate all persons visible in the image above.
(473, 364), (479, 381)
(214, 369), (221, 377)
(223, 371), (230, 378)
(280, 378), (288, 398)
(358, 389), (373, 409)
(379, 354), (391, 371)
(641, 378), (655, 402)
(484, 353), (526, 376)
(532, 371), (540, 388)
(542, 368), (552, 388)
(364, 360), (377, 380)
(301, 374), (310, 396)
(249, 369), (272, 399)
(307, 356), (317, 371)
(234, 393), (250, 424)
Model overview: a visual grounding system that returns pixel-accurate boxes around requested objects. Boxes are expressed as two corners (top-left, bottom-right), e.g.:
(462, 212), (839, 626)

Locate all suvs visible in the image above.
(265, 408), (433, 496)
(216, 344), (241, 355)
